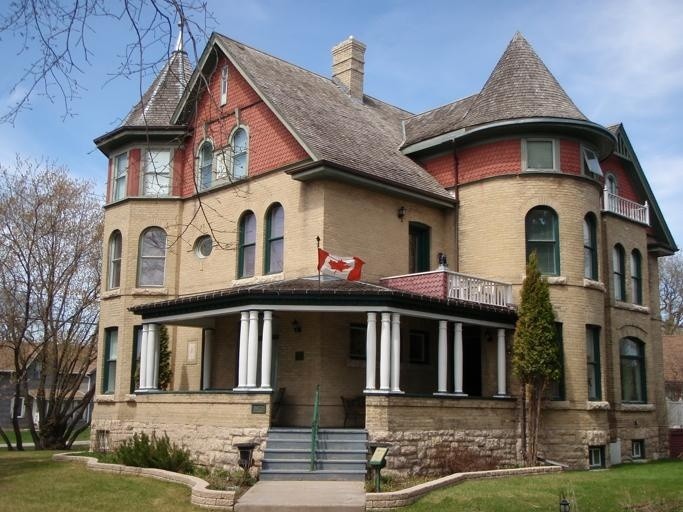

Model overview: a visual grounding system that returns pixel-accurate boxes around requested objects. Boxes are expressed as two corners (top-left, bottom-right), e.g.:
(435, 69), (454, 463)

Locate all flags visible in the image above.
(318, 249), (366, 281)
